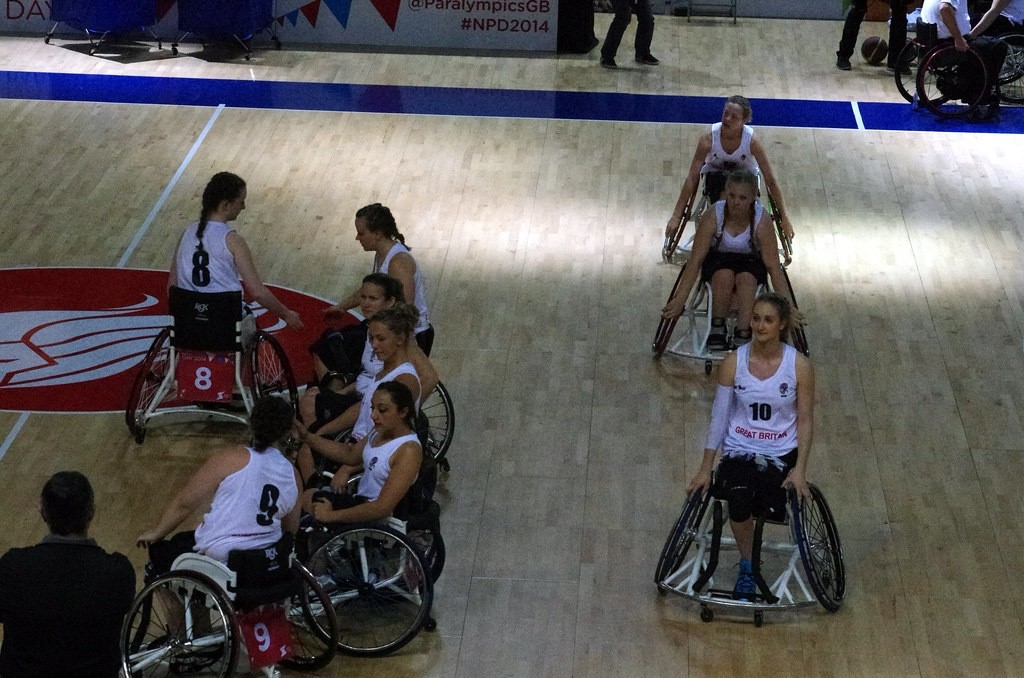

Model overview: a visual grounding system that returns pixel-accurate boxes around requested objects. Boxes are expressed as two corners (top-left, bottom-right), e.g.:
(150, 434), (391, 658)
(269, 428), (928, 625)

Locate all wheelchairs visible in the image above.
(662, 160), (793, 262)
(653, 468), (846, 627)
(125, 285), (301, 454)
(120, 532), (337, 678)
(894, 17), (1024, 118)
(300, 325), (457, 654)
(652, 260), (809, 375)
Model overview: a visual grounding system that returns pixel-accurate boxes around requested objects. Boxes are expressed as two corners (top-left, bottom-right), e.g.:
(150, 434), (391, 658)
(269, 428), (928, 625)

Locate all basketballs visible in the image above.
(861, 36), (888, 63)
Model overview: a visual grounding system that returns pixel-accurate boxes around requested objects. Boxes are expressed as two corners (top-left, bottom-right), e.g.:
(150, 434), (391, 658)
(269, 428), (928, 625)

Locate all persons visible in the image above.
(309, 203), (434, 383)
(919, 0), (1008, 105)
(298, 273), (440, 485)
(660, 171), (808, 350)
(969, 0), (1024, 48)
(0, 472), (136, 678)
(303, 381), (422, 599)
(136, 396), (303, 650)
(685, 294), (814, 602)
(167, 172), (305, 331)
(600, 0), (659, 68)
(836, 0), (912, 75)
(665, 95), (795, 244)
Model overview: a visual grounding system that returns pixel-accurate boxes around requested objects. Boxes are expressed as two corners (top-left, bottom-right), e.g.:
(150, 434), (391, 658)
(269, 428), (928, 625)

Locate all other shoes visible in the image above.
(600, 56), (617, 69)
(635, 52), (659, 65)
(887, 62), (912, 75)
(836, 59), (852, 70)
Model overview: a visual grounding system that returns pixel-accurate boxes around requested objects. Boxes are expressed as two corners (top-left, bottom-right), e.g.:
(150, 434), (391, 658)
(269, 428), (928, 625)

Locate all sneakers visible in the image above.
(707, 317), (727, 350)
(734, 326), (752, 350)
(733, 559), (757, 602)
